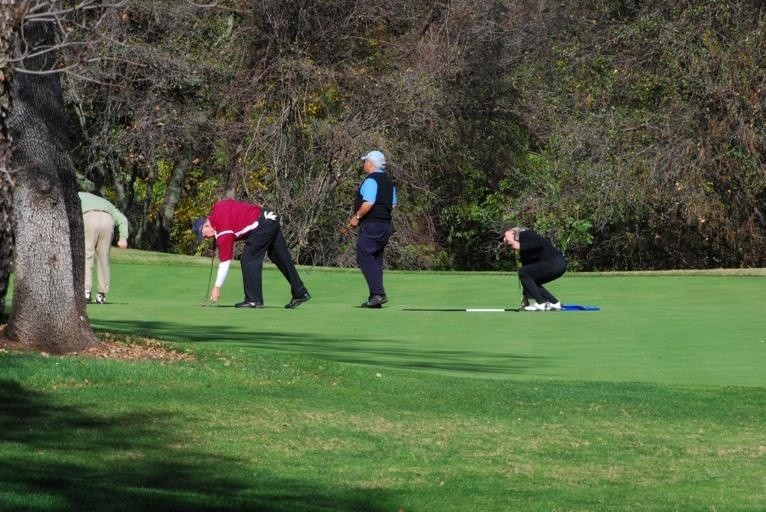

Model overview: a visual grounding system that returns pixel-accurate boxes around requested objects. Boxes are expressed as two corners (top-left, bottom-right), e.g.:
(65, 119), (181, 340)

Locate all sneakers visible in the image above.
(524, 301), (562, 311)
(83, 291), (106, 304)
(361, 295), (390, 308)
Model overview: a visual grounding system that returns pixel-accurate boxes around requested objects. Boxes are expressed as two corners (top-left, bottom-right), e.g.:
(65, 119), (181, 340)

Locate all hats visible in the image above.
(191, 218), (206, 246)
(360, 150), (386, 165)
(496, 223), (518, 240)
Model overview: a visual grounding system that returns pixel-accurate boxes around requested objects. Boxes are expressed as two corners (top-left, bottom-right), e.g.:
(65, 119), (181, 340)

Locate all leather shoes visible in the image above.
(234, 301), (264, 308)
(284, 291), (311, 309)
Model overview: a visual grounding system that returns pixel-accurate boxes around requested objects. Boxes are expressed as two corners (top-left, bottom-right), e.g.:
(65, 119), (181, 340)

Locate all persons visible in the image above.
(496, 223), (567, 311)
(193, 199), (310, 310)
(76, 190), (129, 304)
(349, 151), (398, 307)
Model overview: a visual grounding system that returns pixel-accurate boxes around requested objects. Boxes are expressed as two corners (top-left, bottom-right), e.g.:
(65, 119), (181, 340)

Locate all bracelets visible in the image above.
(354, 213), (362, 220)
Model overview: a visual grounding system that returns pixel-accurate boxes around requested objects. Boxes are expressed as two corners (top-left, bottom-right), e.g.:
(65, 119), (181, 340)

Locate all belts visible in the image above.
(81, 209), (115, 216)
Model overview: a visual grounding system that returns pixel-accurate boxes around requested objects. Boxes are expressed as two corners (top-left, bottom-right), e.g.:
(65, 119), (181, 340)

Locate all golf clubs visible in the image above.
(204, 237), (217, 298)
(303, 233), (340, 276)
